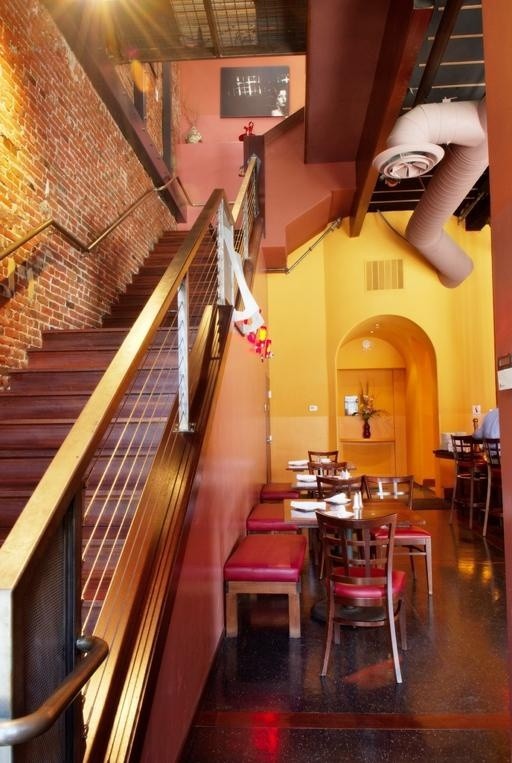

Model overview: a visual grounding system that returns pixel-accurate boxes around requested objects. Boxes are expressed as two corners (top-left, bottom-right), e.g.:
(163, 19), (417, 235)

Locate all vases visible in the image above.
(362, 417), (371, 439)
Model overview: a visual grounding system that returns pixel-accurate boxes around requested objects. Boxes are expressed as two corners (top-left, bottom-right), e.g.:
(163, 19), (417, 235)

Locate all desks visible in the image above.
(283, 498), (426, 611)
(286, 462), (357, 471)
(289, 475), (363, 489)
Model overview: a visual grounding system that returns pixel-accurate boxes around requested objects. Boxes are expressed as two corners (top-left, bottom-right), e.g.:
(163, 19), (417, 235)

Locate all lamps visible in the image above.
(246, 326), (272, 361)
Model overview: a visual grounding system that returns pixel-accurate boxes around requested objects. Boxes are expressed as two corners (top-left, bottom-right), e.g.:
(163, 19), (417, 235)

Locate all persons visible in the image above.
(472, 407), (501, 464)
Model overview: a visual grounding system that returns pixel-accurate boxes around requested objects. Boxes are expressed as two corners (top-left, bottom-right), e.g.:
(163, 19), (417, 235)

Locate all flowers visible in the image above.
(352, 380), (391, 417)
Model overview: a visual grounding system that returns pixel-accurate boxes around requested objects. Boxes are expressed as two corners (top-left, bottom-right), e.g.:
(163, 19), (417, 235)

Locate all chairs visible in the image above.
(364, 474), (431, 596)
(315, 477), (368, 498)
(307, 449), (337, 464)
(308, 462), (348, 474)
(318, 513), (407, 684)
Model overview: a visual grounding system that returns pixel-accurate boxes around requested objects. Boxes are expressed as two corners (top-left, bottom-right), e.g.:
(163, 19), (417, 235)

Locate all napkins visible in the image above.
(327, 493), (349, 501)
(288, 460), (307, 466)
(339, 471), (351, 478)
(290, 501), (326, 510)
(296, 474), (316, 480)
(320, 458), (331, 463)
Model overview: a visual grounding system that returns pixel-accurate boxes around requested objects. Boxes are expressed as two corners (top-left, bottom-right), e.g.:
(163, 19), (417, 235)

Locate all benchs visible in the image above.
(260, 479), (297, 502)
(223, 534), (309, 636)
(247, 503), (302, 536)
